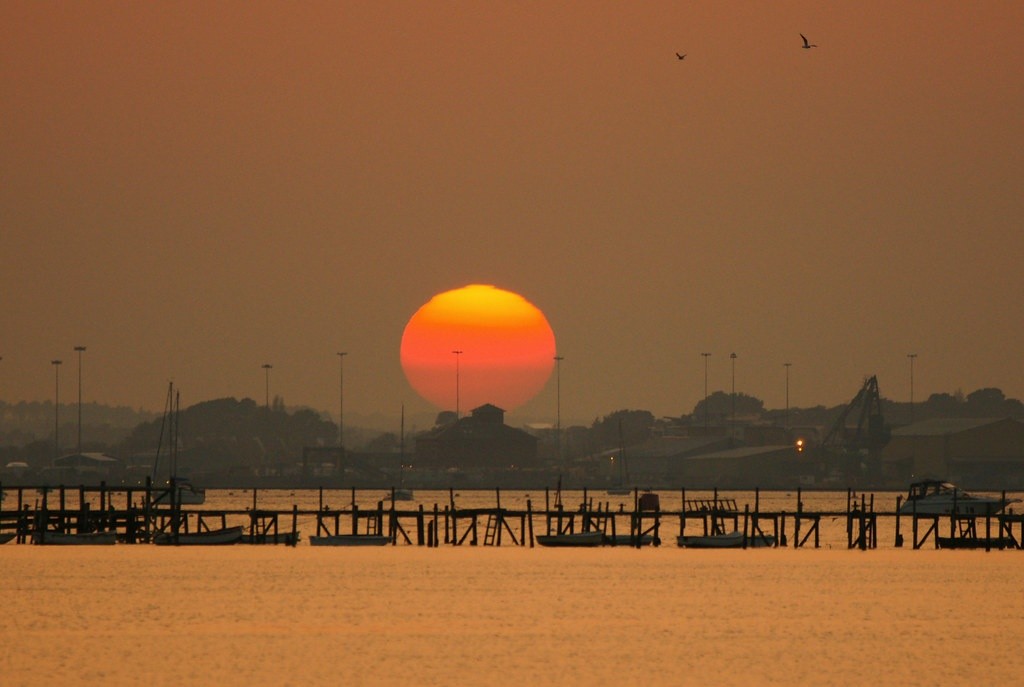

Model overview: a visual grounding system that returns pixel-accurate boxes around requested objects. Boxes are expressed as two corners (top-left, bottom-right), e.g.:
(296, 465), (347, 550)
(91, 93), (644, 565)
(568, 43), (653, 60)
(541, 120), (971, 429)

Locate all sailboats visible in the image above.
(385, 402), (416, 501)
(606, 419), (633, 495)
(146, 378), (209, 504)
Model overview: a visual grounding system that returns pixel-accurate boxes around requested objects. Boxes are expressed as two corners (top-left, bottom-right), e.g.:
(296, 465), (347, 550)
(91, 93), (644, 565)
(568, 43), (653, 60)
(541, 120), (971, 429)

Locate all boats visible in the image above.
(676, 529), (745, 548)
(603, 534), (657, 546)
(241, 530), (302, 547)
(307, 533), (395, 546)
(896, 477), (1024, 518)
(29, 528), (117, 545)
(0, 532), (17, 545)
(741, 534), (777, 548)
(535, 529), (605, 545)
(151, 526), (247, 543)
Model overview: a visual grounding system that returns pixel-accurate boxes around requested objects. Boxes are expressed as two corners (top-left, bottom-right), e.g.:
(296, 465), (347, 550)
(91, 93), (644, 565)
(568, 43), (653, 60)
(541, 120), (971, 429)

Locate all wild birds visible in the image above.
(800, 33), (819, 48)
(676, 52), (687, 60)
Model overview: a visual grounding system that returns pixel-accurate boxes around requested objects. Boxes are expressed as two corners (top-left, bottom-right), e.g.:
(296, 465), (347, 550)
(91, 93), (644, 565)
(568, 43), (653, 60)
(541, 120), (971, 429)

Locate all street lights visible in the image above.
(452, 349), (462, 426)
(906, 354), (917, 426)
(73, 346), (87, 453)
(554, 356), (564, 466)
(730, 351), (740, 448)
(261, 364), (273, 410)
(783, 362), (792, 427)
(701, 352), (712, 442)
(337, 351), (348, 453)
(51, 360), (64, 452)
